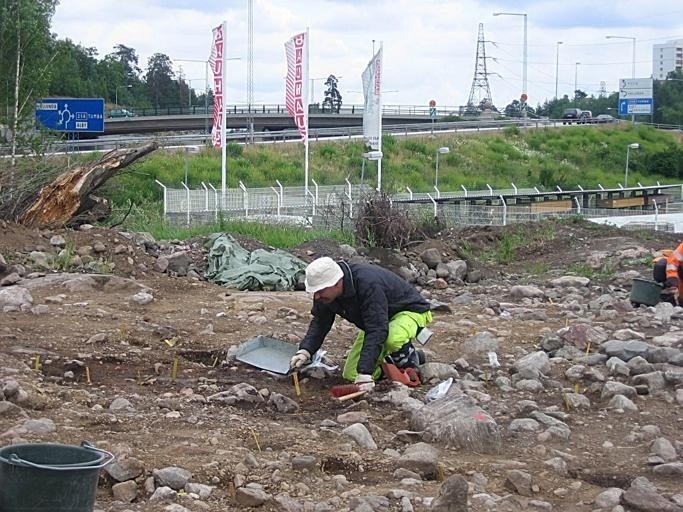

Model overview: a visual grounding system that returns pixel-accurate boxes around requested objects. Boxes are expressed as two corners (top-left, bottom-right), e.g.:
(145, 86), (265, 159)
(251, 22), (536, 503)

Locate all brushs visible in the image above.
(330, 384), (367, 402)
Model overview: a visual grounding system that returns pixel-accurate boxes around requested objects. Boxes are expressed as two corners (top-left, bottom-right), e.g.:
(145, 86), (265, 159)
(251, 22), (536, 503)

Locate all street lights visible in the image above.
(493, 11), (528, 126)
(434, 147), (449, 191)
(283, 74), (345, 108)
(624, 142), (638, 187)
(346, 90), (398, 94)
(173, 56), (244, 133)
(115, 84), (132, 108)
(359, 151), (383, 188)
(606, 35), (636, 126)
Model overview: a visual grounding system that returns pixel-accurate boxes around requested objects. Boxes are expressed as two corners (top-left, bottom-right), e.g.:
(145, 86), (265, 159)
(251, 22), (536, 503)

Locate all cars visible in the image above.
(107, 109), (134, 118)
(561, 108), (613, 125)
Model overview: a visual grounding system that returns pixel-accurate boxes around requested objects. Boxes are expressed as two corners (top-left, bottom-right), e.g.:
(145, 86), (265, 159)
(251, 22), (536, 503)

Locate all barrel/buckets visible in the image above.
(0, 439), (114, 512)
(629, 278), (663, 306)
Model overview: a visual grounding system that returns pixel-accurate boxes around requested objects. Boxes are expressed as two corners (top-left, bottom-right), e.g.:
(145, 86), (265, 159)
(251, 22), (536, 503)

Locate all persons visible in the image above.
(654, 239), (683, 307)
(289, 255), (435, 394)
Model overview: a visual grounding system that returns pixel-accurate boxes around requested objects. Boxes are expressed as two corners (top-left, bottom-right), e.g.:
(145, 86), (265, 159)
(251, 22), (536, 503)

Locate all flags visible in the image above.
(283, 32), (306, 146)
(209, 24), (221, 148)
(360, 48), (379, 150)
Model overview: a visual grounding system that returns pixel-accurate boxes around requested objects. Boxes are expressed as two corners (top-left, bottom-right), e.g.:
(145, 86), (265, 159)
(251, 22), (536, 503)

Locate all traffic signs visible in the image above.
(618, 78), (652, 88)
(618, 89), (653, 97)
(617, 98), (652, 115)
(34, 98), (104, 133)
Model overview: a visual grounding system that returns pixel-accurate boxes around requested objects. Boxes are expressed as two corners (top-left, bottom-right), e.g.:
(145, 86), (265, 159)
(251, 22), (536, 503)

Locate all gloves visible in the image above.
(354, 373), (375, 391)
(290, 349), (311, 369)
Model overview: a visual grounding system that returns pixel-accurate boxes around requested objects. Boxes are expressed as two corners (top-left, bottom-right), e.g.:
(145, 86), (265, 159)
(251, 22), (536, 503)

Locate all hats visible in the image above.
(305, 257), (345, 293)
